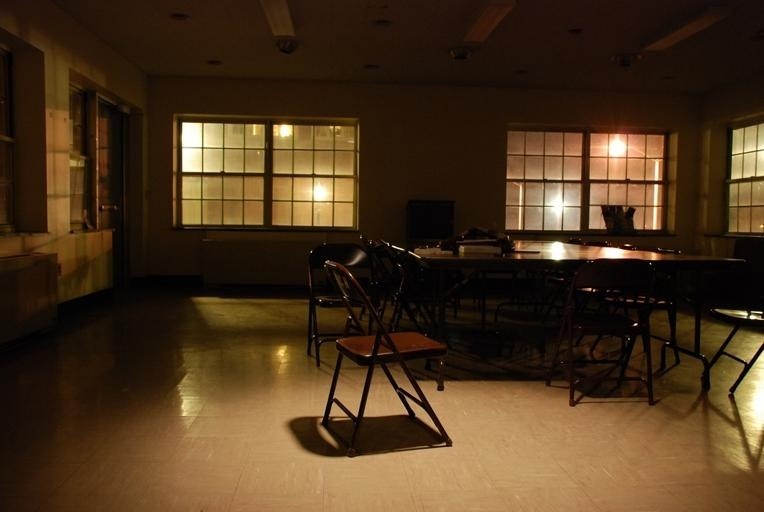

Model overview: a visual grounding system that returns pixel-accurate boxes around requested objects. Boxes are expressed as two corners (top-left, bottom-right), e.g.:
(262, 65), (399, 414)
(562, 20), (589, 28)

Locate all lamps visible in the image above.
(444, 41), (481, 59)
(609, 53), (643, 67)
(272, 35), (299, 54)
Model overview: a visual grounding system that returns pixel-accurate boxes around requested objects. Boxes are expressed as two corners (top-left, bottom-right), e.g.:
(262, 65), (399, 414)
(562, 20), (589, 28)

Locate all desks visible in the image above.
(384, 231), (746, 336)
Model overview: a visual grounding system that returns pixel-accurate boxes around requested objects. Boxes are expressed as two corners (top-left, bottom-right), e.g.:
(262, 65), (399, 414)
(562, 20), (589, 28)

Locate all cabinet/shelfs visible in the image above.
(406, 199), (454, 303)
(200, 238), (327, 290)
(0, 252), (58, 356)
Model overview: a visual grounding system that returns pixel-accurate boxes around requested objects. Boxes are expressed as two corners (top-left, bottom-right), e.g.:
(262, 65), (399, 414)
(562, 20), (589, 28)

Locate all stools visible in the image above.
(707, 297), (764, 401)
(544, 257), (657, 410)
(312, 254), (454, 450)
(355, 233), (442, 337)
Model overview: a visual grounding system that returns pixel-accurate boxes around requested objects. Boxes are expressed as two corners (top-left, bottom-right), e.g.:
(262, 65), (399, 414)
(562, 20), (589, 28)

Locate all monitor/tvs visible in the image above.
(407, 199), (455, 240)
